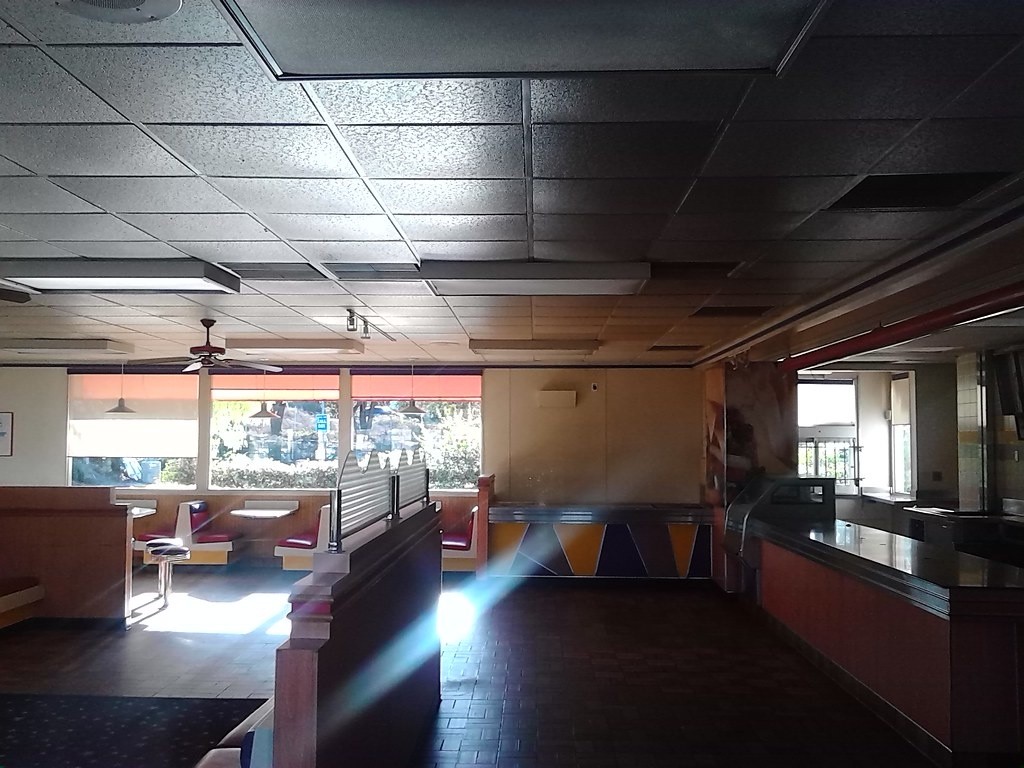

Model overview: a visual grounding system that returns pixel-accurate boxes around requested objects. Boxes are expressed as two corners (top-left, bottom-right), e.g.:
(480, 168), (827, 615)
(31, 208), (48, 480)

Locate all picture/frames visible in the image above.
(0, 411), (13, 457)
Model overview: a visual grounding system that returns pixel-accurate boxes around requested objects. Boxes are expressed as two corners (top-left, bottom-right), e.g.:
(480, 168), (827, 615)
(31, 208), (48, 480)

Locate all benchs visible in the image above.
(274, 504), (331, 571)
(193, 693), (275, 768)
(442, 505), (479, 572)
(133, 500), (244, 566)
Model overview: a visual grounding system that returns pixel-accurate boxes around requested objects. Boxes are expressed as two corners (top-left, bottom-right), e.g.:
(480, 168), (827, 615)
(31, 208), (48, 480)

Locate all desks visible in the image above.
(229, 500), (300, 519)
(116, 498), (157, 517)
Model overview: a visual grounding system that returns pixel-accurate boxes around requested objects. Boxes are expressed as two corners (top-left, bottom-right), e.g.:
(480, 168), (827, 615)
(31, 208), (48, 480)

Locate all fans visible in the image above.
(127, 318), (283, 373)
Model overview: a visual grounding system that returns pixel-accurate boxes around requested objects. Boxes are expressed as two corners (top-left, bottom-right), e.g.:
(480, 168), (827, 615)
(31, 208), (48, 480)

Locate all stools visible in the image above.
(145, 539), (191, 610)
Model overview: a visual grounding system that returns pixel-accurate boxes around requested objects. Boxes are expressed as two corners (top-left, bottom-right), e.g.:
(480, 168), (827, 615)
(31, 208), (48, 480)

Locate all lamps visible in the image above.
(248, 359), (283, 420)
(360, 321), (371, 339)
(106, 356), (136, 415)
(347, 311), (358, 332)
(396, 365), (426, 415)
